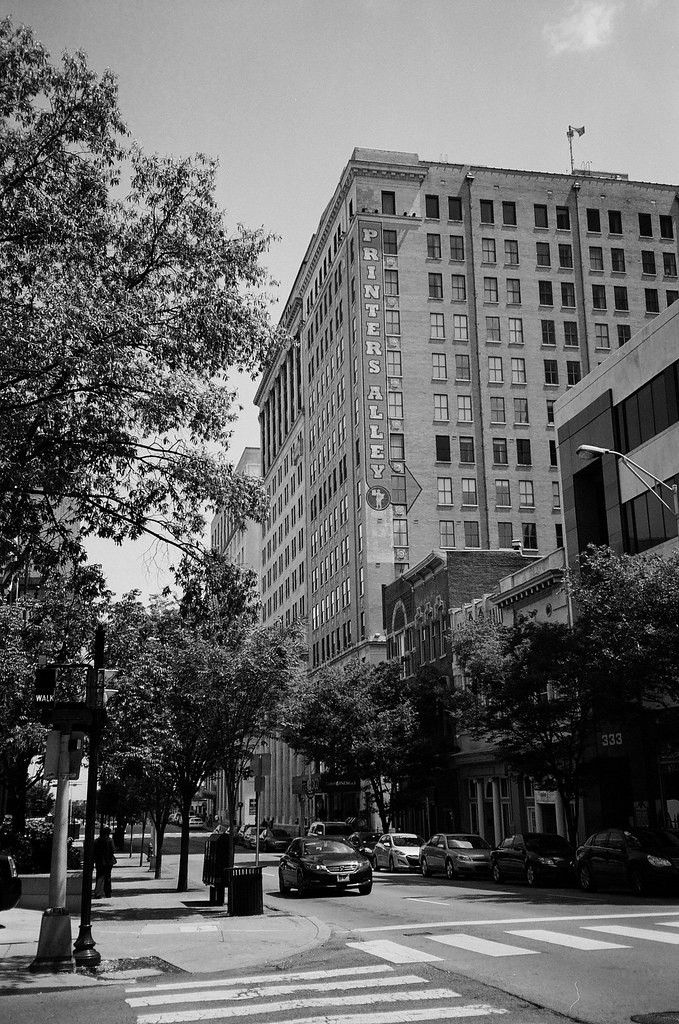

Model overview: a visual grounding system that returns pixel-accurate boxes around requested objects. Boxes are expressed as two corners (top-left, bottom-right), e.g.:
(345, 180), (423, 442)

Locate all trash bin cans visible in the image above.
(224, 865), (268, 917)
(202, 834), (235, 906)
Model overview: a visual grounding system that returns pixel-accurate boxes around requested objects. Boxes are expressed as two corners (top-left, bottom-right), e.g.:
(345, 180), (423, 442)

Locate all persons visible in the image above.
(262, 817), (274, 829)
(94, 827), (119, 899)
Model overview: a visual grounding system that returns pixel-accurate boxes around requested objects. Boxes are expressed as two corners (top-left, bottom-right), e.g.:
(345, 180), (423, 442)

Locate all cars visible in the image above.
(244, 826), (266, 850)
(258, 828), (294, 852)
(189, 817), (204, 829)
(105, 821), (127, 834)
(417, 832), (496, 881)
(345, 830), (384, 861)
(166, 813), (197, 826)
(372, 833), (425, 873)
(574, 826), (679, 897)
(278, 835), (373, 896)
(214, 823), (256, 845)
(489, 833), (578, 887)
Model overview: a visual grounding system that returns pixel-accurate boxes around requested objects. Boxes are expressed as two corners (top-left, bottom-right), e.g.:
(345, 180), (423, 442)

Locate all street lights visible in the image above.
(576, 444), (679, 548)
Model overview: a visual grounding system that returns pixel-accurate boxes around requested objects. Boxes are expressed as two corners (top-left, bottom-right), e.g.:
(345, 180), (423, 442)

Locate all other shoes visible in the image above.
(105, 892), (111, 898)
(95, 893), (102, 898)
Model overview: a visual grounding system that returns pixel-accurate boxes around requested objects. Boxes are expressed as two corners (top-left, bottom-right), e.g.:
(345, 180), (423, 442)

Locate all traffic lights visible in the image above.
(97, 666), (118, 707)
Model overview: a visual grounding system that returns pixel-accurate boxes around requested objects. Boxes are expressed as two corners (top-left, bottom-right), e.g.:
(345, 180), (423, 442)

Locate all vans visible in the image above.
(307, 821), (354, 842)
